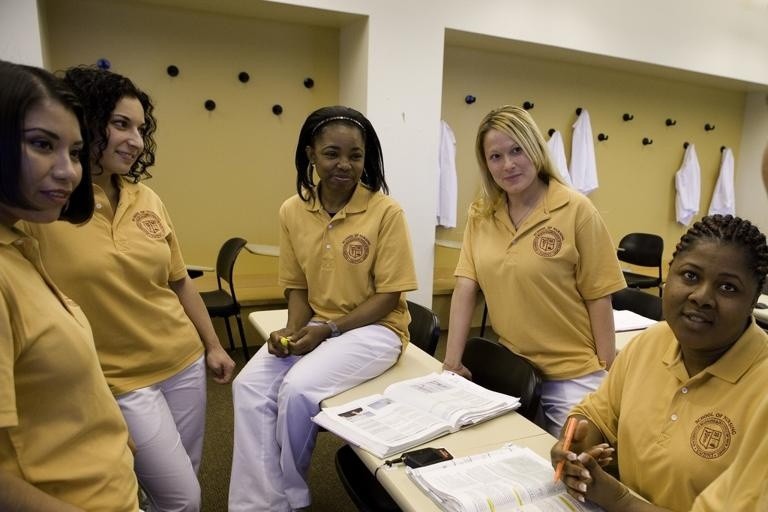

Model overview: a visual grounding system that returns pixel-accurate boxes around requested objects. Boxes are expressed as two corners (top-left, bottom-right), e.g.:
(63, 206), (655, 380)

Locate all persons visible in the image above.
(227, 105), (419, 512)
(551, 213), (768, 512)
(17, 66), (236, 512)
(443, 104), (628, 438)
(0, 59), (142, 512)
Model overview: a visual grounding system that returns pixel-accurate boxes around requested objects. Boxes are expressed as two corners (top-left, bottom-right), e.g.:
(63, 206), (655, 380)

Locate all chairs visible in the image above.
(616, 233), (664, 297)
(613, 288), (663, 321)
(198, 237), (250, 362)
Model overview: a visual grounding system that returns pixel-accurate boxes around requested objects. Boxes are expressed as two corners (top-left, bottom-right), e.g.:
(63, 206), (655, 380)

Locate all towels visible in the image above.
(675, 144), (700, 226)
(546, 130), (572, 188)
(708, 148), (736, 218)
(571, 110), (599, 196)
(435, 120), (458, 229)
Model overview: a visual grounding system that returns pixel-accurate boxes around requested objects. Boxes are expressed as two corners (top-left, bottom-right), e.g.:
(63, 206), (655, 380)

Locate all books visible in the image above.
(611, 309), (658, 333)
(310, 371), (523, 460)
(407, 445), (601, 512)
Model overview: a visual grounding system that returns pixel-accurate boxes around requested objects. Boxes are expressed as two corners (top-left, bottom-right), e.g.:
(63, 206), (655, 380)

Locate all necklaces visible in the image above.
(514, 201), (538, 228)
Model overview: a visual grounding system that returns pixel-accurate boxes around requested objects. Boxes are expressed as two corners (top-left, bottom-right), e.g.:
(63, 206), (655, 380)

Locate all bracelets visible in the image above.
(324, 320), (341, 337)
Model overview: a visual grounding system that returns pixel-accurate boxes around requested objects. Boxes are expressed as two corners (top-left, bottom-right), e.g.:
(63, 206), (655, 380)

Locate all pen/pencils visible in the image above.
(554, 417), (578, 485)
